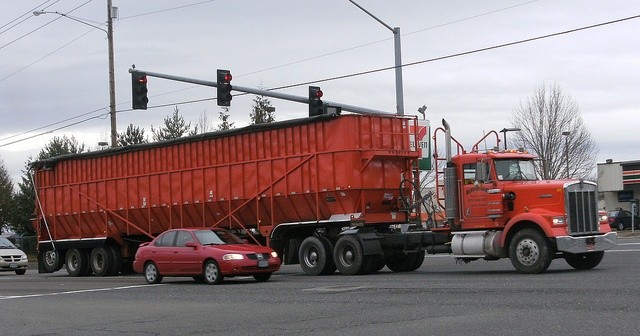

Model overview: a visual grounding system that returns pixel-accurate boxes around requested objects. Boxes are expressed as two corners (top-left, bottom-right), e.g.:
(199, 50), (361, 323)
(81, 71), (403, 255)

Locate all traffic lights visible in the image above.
(309, 85), (323, 117)
(132, 71), (148, 109)
(217, 69), (232, 106)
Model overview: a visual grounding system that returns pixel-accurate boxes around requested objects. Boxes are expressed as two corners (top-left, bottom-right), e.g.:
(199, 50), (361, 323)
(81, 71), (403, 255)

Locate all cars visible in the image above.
(607, 209), (640, 230)
(132, 227), (282, 285)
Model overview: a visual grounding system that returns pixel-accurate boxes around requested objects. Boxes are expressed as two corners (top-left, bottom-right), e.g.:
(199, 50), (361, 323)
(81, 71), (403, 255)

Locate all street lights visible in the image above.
(561, 129), (573, 179)
(499, 127), (521, 150)
(32, 9), (116, 147)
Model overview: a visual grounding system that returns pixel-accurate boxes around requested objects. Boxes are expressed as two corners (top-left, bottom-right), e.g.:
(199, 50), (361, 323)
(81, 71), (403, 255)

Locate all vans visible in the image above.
(0, 237), (28, 275)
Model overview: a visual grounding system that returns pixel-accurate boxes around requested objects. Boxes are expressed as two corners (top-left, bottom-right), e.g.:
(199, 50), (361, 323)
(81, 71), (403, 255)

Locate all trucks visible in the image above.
(26, 115), (619, 277)
